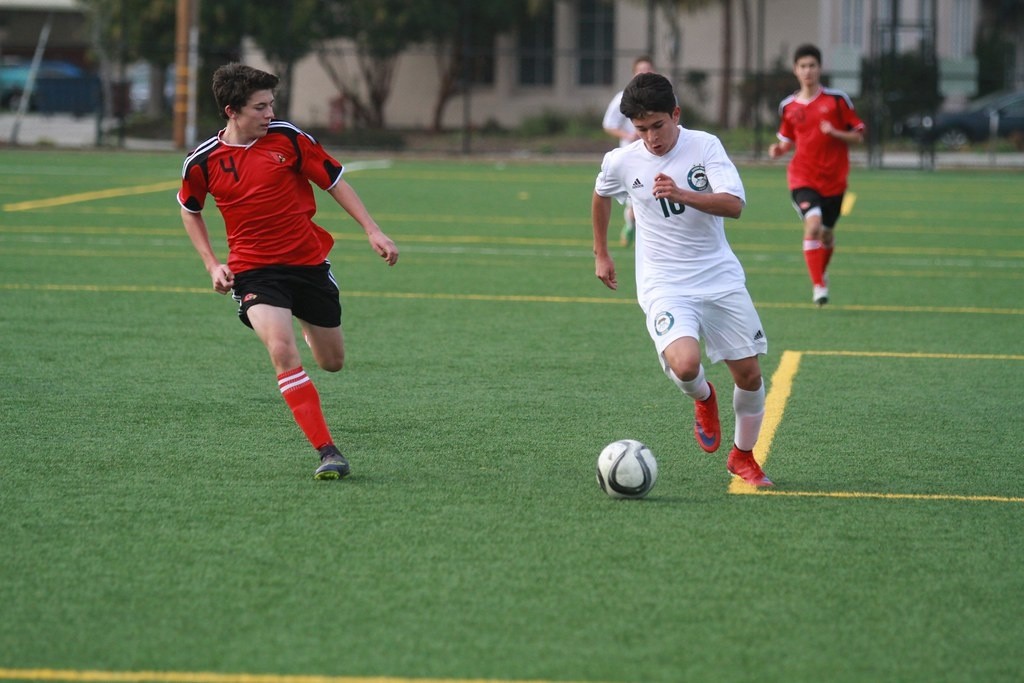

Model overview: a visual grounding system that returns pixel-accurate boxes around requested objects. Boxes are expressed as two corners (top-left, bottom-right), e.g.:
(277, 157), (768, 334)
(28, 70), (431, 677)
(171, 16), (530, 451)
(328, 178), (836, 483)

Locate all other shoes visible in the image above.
(812, 283), (828, 304)
(620, 225), (635, 246)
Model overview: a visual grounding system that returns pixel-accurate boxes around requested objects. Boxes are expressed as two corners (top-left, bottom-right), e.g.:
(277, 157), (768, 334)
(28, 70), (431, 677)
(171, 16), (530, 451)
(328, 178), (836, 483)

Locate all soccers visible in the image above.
(594, 438), (659, 500)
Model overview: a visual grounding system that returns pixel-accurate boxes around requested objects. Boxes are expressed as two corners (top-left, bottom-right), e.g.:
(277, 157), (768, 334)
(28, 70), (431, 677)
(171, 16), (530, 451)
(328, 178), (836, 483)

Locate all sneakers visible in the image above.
(313, 445), (349, 480)
(694, 380), (721, 452)
(727, 448), (775, 487)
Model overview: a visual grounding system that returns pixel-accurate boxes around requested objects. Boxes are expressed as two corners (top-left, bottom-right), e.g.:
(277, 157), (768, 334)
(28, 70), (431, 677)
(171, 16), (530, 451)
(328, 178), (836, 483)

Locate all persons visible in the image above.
(590, 74), (772, 486)
(768, 44), (866, 304)
(176, 63), (400, 479)
(601, 56), (657, 247)
